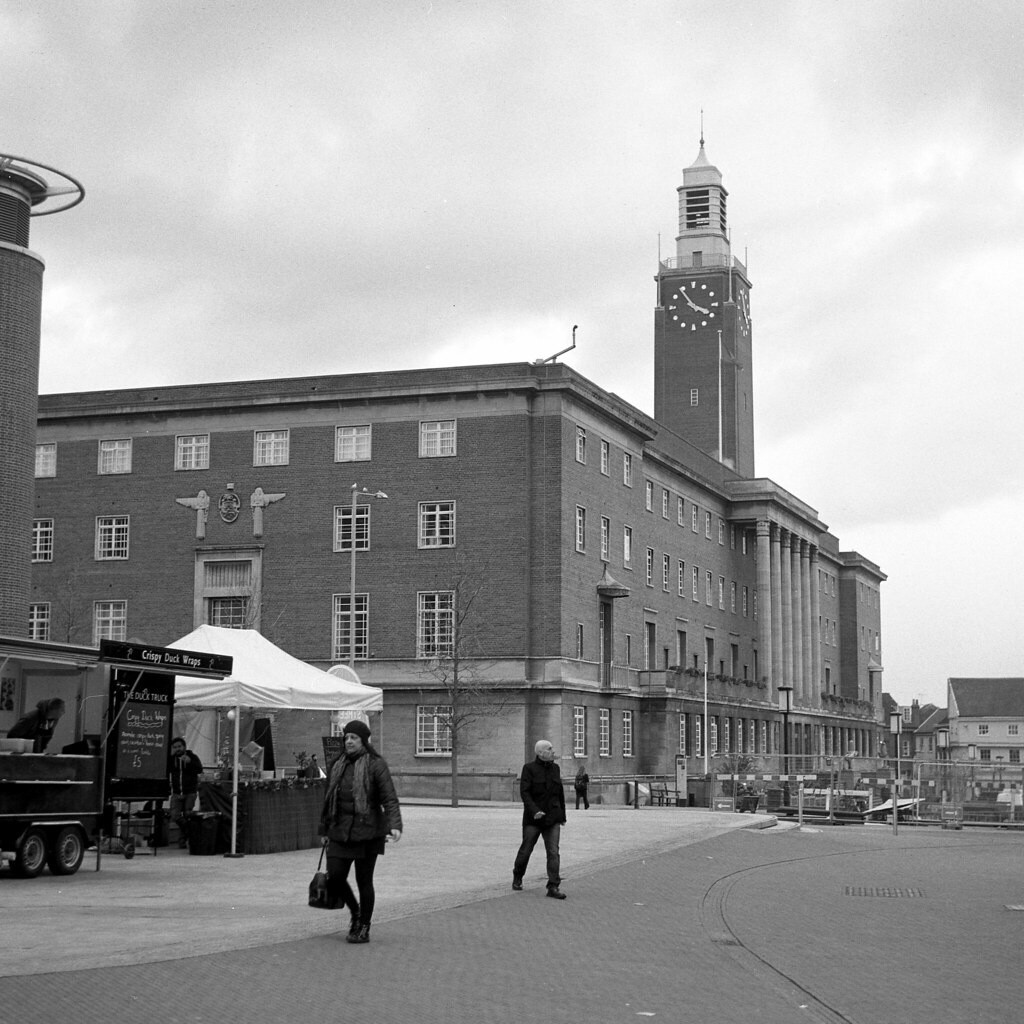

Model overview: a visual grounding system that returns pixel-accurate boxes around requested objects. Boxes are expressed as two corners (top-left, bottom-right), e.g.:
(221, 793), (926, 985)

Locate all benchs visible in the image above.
(650, 782), (681, 806)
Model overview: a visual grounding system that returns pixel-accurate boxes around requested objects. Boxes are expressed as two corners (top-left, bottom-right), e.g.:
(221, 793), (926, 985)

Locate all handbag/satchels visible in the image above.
(309, 872), (345, 909)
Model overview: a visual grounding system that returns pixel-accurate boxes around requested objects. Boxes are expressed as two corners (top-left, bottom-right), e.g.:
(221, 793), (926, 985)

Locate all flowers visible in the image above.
(214, 749), (234, 771)
(292, 749), (311, 769)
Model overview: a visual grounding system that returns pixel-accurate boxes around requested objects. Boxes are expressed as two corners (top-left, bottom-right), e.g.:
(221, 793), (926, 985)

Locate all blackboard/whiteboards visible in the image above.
(322, 736), (345, 774)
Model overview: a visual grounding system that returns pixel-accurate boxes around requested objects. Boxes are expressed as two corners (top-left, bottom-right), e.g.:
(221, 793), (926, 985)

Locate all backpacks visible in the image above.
(575, 780), (583, 789)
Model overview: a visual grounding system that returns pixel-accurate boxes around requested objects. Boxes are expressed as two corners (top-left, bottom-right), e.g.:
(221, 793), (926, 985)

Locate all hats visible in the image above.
(344, 720), (372, 738)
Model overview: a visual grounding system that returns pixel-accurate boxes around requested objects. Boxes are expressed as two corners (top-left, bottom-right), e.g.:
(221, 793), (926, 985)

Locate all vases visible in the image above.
(220, 772), (230, 780)
(297, 770), (314, 779)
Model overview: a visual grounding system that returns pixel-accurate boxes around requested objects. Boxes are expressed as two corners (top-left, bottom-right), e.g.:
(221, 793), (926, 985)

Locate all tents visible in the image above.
(117, 624), (383, 857)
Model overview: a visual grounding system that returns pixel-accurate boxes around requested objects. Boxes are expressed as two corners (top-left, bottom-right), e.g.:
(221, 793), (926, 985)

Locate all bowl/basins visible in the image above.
(261, 770), (274, 779)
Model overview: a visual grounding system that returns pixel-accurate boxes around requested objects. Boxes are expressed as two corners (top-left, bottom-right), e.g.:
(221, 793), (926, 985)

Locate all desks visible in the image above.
(199, 778), (329, 855)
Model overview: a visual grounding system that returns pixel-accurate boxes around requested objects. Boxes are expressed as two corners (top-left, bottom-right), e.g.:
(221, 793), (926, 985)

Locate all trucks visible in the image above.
(0, 635), (112, 880)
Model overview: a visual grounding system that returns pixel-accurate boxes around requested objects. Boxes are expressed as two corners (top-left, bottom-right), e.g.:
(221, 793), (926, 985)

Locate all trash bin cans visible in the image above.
(183, 811), (223, 855)
(628, 782), (649, 806)
(765, 787), (783, 812)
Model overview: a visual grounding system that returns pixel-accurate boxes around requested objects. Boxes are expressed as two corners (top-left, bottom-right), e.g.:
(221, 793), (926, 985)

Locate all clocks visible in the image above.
(738, 289), (750, 338)
(668, 278), (720, 332)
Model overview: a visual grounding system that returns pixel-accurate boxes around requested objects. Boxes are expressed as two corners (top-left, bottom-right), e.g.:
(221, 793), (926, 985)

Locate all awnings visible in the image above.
(862, 798), (925, 815)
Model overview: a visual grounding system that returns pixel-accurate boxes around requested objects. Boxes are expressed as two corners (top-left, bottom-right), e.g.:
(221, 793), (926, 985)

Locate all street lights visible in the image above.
(347, 482), (389, 666)
(777, 686), (794, 817)
(890, 709), (902, 797)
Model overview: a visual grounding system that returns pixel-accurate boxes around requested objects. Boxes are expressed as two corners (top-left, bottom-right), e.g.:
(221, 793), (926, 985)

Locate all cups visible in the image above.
(275, 768), (284, 778)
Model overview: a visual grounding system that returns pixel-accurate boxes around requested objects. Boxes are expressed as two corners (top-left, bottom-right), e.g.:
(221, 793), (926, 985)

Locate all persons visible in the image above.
(169, 737), (203, 850)
(574, 765), (589, 810)
(512, 740), (567, 900)
(308, 721), (402, 944)
(7, 697), (65, 753)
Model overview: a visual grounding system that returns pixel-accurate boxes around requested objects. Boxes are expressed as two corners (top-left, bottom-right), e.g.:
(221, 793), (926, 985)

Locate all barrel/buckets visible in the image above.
(767, 789), (784, 807)
(185, 811), (222, 855)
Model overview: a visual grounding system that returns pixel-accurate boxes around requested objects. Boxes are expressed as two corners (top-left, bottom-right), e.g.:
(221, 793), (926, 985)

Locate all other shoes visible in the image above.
(576, 807), (579, 809)
(585, 804), (590, 810)
(512, 875), (523, 890)
(547, 888), (567, 900)
(178, 841), (186, 849)
(346, 910), (371, 944)
